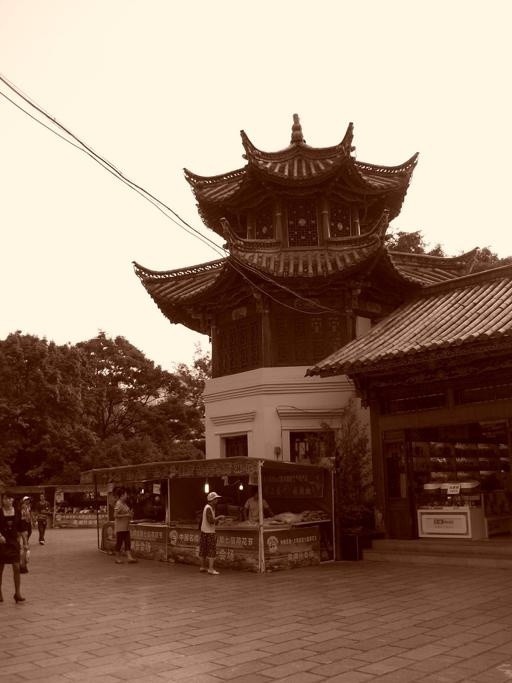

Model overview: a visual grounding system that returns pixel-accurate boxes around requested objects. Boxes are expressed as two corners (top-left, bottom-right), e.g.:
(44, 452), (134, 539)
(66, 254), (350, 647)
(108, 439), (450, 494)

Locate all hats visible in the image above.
(207, 491), (222, 501)
(23, 496), (29, 501)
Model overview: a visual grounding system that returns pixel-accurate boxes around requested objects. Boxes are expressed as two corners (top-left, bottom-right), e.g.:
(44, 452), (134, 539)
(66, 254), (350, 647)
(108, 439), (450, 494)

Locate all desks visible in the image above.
(30, 512), (320, 574)
(342, 531), (377, 560)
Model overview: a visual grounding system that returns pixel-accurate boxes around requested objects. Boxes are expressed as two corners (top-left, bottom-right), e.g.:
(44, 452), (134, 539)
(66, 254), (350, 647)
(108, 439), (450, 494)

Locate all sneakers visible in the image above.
(199, 567), (219, 574)
(115, 558), (138, 564)
(40, 540), (45, 544)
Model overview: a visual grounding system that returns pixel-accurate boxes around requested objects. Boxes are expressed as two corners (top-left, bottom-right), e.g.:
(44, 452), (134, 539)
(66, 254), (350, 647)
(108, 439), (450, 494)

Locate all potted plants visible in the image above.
(319, 398), (378, 535)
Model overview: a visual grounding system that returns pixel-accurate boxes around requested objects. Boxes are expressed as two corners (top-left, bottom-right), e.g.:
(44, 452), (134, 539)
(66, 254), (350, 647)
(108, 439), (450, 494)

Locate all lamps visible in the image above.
(204, 477), (209, 494)
(238, 476), (244, 490)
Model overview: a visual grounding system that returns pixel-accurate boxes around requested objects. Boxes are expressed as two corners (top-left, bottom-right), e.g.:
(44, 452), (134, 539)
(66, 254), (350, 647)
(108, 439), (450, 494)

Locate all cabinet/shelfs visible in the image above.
(388, 438), (512, 539)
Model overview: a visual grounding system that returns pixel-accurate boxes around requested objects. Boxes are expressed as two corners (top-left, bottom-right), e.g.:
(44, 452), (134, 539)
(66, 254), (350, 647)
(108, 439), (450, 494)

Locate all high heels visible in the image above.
(0, 591), (26, 604)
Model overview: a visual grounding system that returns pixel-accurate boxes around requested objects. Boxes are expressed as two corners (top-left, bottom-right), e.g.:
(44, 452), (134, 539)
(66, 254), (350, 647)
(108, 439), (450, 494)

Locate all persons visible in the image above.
(0, 490), (27, 606)
(198, 490), (224, 575)
(37, 490), (51, 544)
(112, 488), (139, 563)
(241, 489), (277, 521)
(18, 494), (37, 543)
